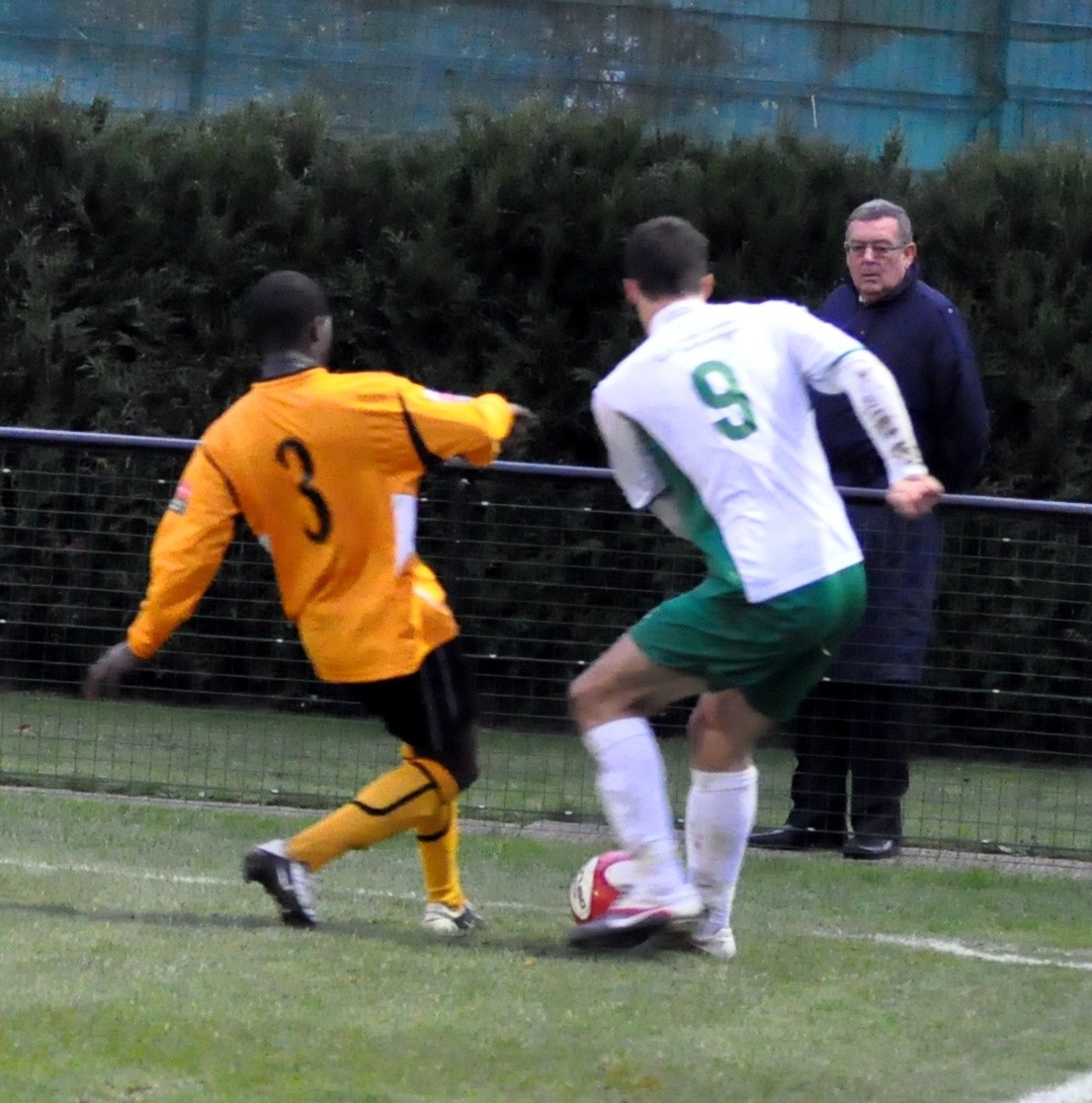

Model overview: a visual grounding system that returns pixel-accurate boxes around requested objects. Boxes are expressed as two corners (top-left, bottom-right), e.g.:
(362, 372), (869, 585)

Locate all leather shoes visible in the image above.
(845, 831), (902, 857)
(749, 824), (845, 851)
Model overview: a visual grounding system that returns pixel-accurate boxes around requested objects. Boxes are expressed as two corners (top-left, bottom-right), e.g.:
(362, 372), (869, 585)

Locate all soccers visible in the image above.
(569, 851), (647, 925)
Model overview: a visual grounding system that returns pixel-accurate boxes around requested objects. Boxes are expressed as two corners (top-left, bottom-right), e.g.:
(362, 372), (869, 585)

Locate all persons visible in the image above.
(83, 274), (541, 948)
(742, 199), (978, 855)
(569, 222), (946, 956)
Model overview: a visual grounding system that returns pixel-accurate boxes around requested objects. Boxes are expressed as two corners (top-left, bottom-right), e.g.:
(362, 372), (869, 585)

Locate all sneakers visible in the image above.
(674, 928), (737, 958)
(240, 840), (323, 931)
(417, 902), (487, 935)
(566, 883), (703, 949)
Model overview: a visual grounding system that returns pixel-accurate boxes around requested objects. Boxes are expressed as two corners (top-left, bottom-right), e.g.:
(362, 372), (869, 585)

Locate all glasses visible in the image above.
(848, 244), (905, 254)
